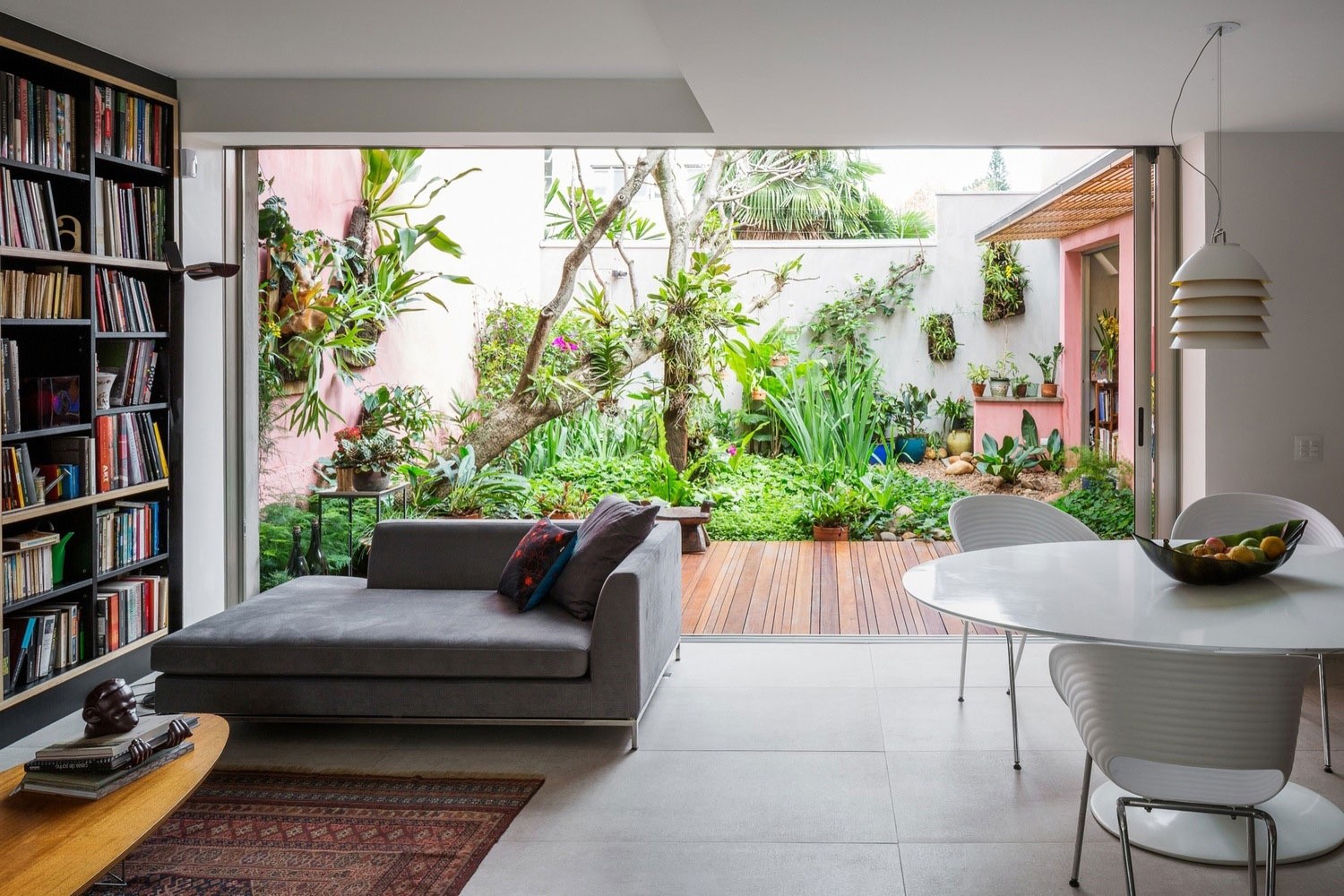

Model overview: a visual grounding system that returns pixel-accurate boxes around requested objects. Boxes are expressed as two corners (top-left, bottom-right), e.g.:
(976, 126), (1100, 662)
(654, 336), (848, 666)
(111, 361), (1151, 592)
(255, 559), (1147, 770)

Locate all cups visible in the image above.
(96, 371), (118, 410)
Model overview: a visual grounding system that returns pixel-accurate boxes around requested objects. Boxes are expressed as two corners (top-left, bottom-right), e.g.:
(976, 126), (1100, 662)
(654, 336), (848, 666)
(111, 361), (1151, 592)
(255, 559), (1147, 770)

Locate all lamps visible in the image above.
(1169, 22), (1274, 350)
(141, 241), (241, 709)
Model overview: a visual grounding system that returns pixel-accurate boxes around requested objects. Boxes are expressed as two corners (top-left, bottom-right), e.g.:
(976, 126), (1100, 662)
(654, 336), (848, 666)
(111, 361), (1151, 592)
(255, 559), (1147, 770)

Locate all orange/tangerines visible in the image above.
(1192, 535), (1286, 563)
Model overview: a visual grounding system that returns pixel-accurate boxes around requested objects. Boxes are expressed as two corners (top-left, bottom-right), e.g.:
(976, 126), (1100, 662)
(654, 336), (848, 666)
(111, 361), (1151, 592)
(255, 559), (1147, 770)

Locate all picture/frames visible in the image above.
(1089, 350), (1119, 383)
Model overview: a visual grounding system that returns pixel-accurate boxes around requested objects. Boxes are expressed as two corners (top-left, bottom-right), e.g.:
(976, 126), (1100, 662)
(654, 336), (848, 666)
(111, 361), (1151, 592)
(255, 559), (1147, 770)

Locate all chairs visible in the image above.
(1169, 493), (1344, 773)
(1049, 642), (1321, 896)
(948, 494), (1103, 770)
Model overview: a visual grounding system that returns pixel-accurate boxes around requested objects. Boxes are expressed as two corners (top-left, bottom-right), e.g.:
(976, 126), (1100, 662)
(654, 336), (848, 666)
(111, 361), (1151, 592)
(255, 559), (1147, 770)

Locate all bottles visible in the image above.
(304, 518), (330, 576)
(287, 525), (310, 578)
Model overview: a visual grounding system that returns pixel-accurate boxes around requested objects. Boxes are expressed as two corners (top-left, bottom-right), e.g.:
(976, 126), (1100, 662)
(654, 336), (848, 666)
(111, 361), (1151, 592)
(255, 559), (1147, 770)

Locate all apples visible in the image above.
(1239, 538), (1259, 548)
(1248, 547), (1267, 562)
(1205, 537), (1226, 553)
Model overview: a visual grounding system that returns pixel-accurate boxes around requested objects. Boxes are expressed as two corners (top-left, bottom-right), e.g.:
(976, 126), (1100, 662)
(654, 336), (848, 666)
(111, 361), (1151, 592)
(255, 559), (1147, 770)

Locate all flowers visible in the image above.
(1093, 307), (1119, 377)
(331, 428), (361, 468)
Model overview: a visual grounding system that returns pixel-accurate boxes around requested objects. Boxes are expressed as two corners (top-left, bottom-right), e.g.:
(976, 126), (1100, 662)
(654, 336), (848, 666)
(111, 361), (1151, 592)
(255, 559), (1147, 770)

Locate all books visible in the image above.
(0, 59), (201, 804)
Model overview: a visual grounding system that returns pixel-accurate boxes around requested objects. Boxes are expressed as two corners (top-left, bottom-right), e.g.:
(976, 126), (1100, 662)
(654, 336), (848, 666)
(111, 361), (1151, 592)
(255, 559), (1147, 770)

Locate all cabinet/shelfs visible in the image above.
(0, 37), (183, 746)
(1095, 382), (1119, 462)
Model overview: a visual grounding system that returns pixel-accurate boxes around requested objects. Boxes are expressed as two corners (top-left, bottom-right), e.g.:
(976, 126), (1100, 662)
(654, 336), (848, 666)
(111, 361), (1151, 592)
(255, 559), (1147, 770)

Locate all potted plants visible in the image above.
(864, 381), (974, 463)
(1060, 446), (1134, 492)
(341, 433), (404, 492)
(965, 342), (1064, 398)
(802, 480), (873, 541)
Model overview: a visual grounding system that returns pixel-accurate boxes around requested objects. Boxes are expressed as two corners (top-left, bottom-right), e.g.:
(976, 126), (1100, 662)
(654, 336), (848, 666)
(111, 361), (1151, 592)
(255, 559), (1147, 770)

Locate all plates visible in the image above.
(1131, 519), (1308, 586)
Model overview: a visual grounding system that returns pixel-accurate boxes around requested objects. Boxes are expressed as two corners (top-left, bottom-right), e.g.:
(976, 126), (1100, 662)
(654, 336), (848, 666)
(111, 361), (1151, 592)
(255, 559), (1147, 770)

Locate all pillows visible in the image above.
(551, 493), (660, 620)
(497, 515), (578, 613)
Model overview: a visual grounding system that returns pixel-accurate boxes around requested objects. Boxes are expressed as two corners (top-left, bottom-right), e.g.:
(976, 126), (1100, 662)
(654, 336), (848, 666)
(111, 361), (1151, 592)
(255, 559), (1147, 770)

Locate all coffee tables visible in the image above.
(0, 712), (229, 896)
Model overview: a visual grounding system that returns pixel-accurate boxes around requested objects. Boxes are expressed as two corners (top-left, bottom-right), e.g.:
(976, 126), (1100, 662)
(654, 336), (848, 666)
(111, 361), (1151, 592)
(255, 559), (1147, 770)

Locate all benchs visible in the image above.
(150, 518), (681, 750)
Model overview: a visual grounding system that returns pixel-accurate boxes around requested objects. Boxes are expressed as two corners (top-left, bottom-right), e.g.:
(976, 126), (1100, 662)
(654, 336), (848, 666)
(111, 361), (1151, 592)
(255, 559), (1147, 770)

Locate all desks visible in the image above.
(315, 481), (409, 577)
(903, 540), (1344, 866)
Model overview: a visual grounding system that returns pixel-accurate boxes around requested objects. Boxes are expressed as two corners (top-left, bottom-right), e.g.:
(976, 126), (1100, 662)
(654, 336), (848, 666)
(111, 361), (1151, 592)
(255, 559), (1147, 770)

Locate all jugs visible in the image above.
(35, 518), (75, 583)
(33, 467), (72, 503)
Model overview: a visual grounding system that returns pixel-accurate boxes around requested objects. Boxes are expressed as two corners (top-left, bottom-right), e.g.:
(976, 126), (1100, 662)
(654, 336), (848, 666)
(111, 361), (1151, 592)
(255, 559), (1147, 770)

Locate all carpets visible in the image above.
(81, 762), (547, 896)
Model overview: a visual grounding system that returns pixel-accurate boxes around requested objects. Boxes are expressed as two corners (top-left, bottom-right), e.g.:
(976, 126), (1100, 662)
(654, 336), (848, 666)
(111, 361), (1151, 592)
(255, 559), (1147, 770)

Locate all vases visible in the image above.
(1106, 349), (1116, 382)
(336, 468), (356, 492)
